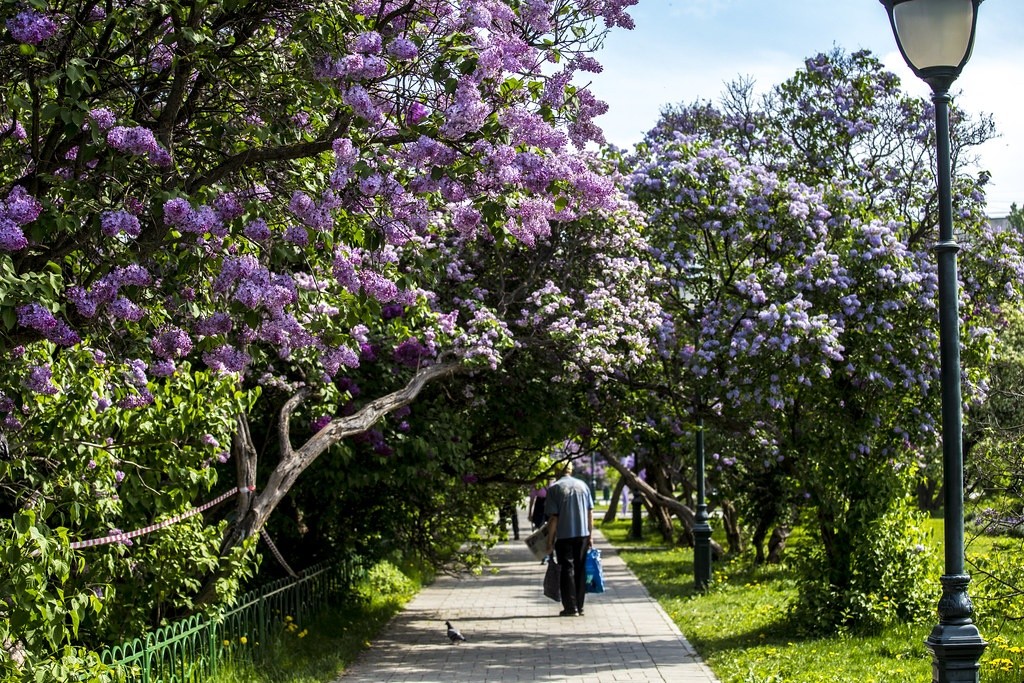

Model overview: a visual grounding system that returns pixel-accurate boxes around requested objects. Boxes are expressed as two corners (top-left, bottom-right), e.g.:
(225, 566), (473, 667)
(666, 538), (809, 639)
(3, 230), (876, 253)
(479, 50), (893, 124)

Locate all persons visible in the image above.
(529, 479), (556, 565)
(546, 459), (594, 617)
(498, 489), (523, 541)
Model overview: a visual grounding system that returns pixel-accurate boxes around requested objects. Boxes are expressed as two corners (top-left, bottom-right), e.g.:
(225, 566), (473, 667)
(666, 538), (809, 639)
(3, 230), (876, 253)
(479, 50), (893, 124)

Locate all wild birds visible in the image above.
(445, 621), (466, 645)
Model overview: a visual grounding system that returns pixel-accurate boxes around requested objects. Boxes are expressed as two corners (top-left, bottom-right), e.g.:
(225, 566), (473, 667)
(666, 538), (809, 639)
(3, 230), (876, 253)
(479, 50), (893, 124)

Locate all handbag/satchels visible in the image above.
(585, 547), (605, 593)
(544, 551), (561, 602)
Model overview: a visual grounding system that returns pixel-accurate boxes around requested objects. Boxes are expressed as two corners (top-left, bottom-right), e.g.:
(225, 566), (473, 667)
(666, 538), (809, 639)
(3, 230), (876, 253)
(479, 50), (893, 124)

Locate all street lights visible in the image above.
(882, 0), (988, 683)
(678, 268), (715, 591)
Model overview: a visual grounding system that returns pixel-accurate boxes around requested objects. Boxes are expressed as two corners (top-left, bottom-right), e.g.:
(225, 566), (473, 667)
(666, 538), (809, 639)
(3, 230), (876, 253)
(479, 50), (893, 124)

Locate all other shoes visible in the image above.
(578, 607), (584, 615)
(560, 606), (579, 617)
